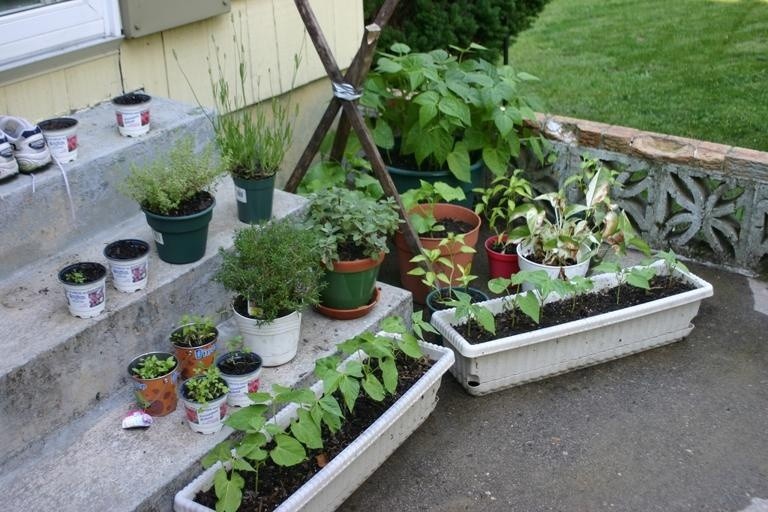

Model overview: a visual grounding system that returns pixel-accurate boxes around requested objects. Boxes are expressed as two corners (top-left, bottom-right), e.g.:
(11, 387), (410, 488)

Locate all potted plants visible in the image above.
(177, 362), (229, 434)
(407, 230), (490, 345)
(171, 8), (305, 225)
(127, 351), (179, 417)
(471, 168), (536, 289)
(431, 248), (714, 396)
(117, 133), (233, 264)
(505, 166), (652, 295)
(173, 310), (456, 512)
(392, 179), (481, 305)
(297, 187), (405, 320)
(169, 313), (219, 381)
(210, 214), (330, 367)
(356, 41), (553, 210)
(215, 334), (263, 408)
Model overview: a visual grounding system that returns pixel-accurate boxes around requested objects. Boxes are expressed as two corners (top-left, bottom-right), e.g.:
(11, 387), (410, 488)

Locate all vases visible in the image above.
(102, 238), (151, 295)
(38, 117), (80, 165)
(56, 262), (107, 318)
(111, 91), (154, 136)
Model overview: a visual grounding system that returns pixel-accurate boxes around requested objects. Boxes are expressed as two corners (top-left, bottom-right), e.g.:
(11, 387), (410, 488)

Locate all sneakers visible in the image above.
(0, 116), (52, 184)
